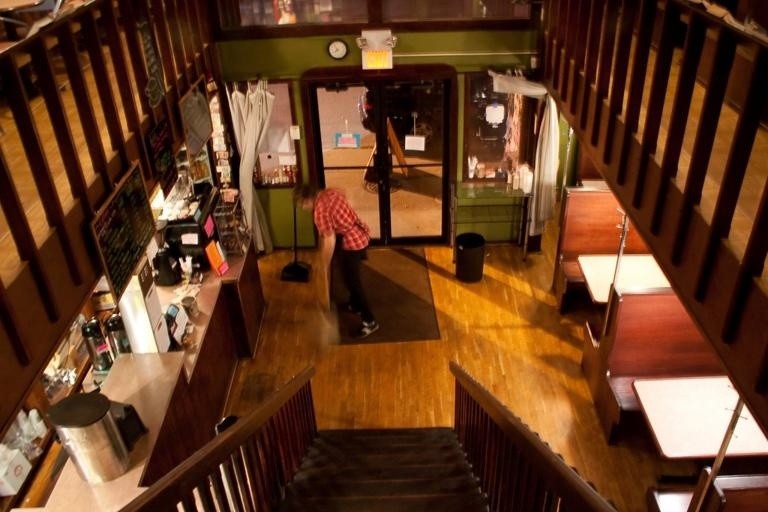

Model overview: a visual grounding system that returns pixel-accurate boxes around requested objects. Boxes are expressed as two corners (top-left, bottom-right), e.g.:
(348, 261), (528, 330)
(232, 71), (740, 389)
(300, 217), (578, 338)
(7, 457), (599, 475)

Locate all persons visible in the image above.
(357, 79), (402, 194)
(291, 182), (380, 339)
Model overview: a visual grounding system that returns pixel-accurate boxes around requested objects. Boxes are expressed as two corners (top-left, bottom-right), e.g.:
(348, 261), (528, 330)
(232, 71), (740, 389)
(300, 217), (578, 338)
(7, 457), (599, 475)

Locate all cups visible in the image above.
(469, 163), (486, 179)
(181, 296), (200, 319)
(15, 408), (47, 438)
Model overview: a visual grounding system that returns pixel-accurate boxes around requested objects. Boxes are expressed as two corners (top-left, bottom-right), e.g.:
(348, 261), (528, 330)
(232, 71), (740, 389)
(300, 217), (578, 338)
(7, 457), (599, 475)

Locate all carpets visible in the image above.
(330, 247), (440, 346)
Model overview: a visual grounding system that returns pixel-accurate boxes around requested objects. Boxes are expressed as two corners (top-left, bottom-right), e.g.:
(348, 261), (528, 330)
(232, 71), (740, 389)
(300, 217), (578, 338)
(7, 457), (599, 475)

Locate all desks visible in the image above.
(577, 253), (671, 305)
(631, 376), (767, 461)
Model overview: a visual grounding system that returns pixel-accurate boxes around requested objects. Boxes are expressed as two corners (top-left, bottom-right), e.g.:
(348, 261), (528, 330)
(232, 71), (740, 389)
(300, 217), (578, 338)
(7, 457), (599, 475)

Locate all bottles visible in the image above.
(81, 316), (133, 372)
(274, 169), (280, 184)
(512, 174), (520, 190)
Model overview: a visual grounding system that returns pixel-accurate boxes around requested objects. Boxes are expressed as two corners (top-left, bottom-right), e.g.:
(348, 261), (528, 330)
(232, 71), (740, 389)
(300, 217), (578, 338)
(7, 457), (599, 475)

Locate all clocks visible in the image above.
(327, 39), (349, 60)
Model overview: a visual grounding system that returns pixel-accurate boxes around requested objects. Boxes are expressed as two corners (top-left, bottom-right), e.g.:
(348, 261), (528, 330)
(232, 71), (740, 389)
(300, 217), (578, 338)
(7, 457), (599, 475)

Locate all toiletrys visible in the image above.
(513, 169), (519, 190)
(495, 167), (506, 179)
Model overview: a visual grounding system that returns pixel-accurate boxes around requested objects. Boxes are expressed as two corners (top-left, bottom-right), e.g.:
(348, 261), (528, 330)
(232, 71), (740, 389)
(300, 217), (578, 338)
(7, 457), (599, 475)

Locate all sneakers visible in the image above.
(339, 303), (361, 315)
(358, 321), (379, 339)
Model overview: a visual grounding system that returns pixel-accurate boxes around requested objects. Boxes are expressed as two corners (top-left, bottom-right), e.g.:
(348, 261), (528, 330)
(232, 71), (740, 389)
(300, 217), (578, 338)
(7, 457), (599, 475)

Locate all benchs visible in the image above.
(542, 183), (651, 316)
(645, 467), (767, 512)
(583, 281), (730, 445)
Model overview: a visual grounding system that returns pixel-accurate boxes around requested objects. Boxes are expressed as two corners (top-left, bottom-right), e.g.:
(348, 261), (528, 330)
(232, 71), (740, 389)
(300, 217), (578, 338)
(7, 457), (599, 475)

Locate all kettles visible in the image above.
(152, 247), (181, 287)
